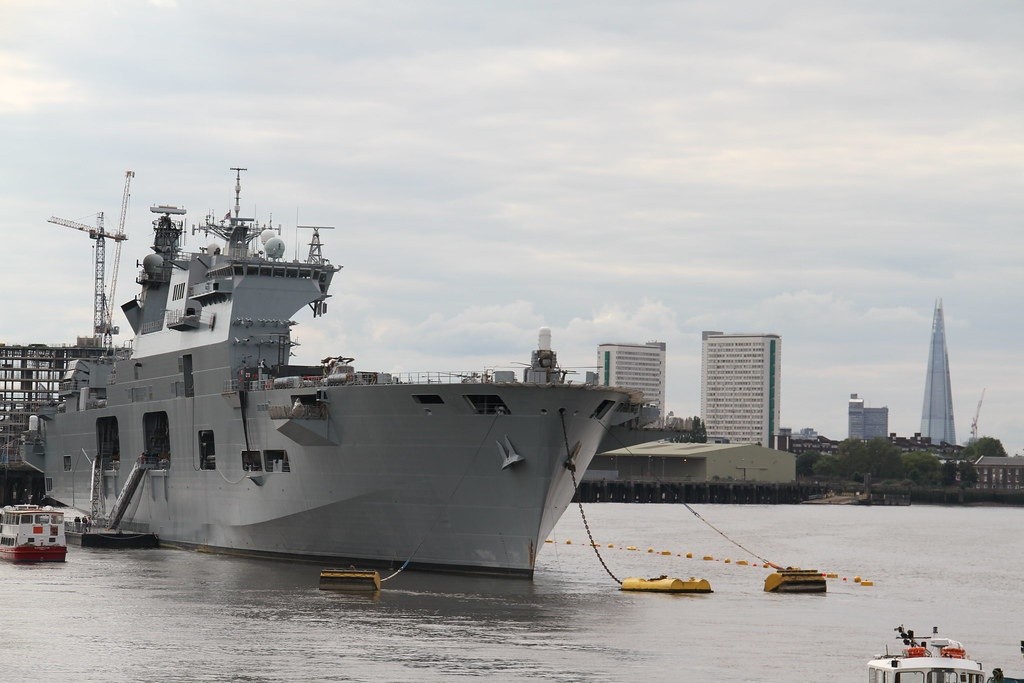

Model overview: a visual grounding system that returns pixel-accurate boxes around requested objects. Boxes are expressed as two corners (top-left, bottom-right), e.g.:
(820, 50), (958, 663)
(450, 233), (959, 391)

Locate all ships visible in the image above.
(19, 163), (643, 582)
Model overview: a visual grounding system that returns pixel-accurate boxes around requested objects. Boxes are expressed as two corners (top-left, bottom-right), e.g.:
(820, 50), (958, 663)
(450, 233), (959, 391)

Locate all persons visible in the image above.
(140, 452), (144, 464)
(145, 450), (149, 464)
(87, 516), (92, 532)
(82, 516), (87, 531)
(261, 358), (267, 368)
(74, 517), (81, 530)
(95, 452), (100, 468)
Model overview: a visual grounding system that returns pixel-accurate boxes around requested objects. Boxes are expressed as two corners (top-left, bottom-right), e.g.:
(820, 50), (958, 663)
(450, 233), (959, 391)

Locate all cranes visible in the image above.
(103, 168), (138, 356)
(970, 387), (986, 435)
(46, 209), (128, 351)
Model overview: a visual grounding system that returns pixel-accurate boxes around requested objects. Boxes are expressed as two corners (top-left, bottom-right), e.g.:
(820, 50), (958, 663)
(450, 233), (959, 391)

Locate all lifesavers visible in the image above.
(941, 648), (965, 658)
(540, 355), (553, 368)
(264, 380), (273, 390)
(904, 647), (928, 657)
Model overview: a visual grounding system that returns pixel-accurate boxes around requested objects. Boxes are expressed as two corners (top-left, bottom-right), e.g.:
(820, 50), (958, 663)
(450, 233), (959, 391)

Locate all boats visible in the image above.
(0, 503), (71, 563)
(867, 627), (983, 683)
